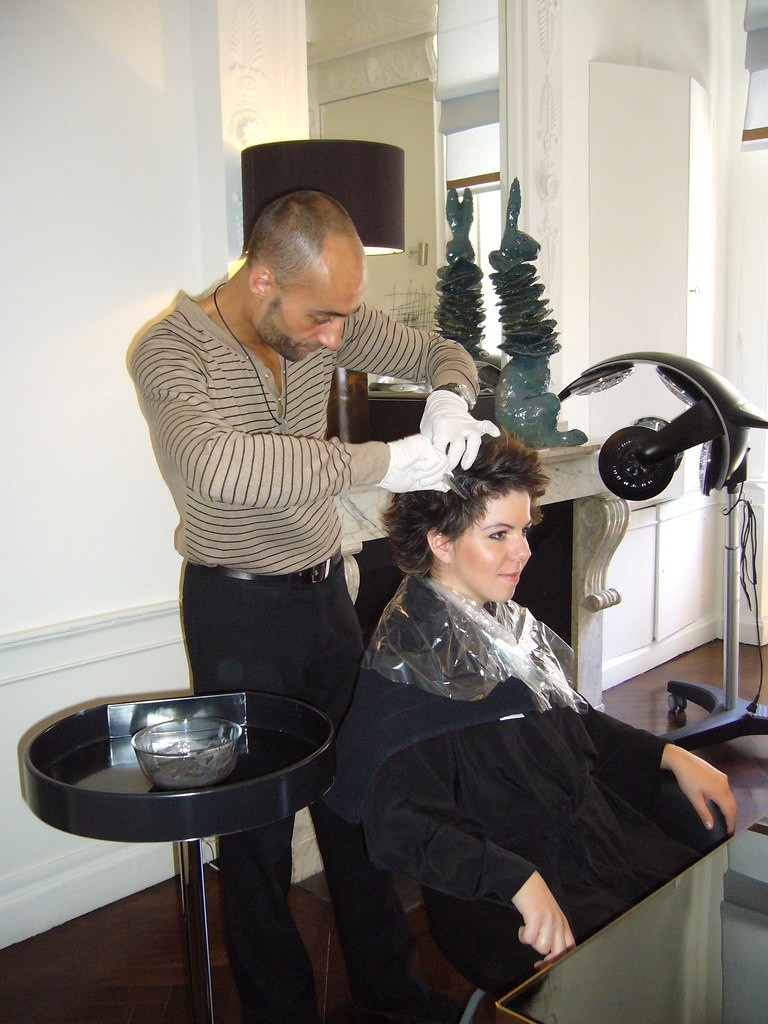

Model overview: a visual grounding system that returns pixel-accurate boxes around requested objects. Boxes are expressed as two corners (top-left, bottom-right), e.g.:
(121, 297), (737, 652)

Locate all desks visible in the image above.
(24, 691), (339, 1023)
(495, 808), (768, 1024)
(332, 436), (630, 715)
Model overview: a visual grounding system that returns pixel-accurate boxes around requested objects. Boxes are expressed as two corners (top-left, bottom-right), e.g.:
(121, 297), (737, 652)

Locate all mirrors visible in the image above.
(220, 1), (508, 399)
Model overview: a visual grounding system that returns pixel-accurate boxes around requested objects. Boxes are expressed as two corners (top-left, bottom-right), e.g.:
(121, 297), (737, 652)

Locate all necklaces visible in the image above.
(214, 283), (287, 431)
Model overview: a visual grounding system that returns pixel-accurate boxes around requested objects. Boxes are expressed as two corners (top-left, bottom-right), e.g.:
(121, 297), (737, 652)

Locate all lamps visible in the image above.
(241, 140), (405, 441)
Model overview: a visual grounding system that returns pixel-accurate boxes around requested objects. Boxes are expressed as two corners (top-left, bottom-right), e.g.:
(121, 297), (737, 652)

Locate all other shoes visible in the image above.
(349, 990), (463, 1024)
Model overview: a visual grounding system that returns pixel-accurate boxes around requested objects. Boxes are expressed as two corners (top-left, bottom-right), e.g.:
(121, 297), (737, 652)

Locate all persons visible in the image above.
(323, 428), (738, 991)
(131, 190), (503, 1024)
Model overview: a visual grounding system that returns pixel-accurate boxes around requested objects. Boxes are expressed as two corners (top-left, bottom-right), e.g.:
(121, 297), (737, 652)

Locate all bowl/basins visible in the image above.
(131, 717), (243, 791)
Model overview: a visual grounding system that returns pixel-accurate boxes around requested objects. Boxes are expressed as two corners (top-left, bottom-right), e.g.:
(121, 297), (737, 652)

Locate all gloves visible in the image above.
(419, 390), (501, 470)
(374, 434), (454, 493)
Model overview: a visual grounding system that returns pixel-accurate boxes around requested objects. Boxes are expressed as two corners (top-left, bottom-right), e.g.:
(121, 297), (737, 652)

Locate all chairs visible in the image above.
(392, 745), (727, 1024)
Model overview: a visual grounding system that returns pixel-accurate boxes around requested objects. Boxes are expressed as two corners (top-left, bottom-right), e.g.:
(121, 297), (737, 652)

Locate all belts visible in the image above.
(201, 544), (341, 584)
